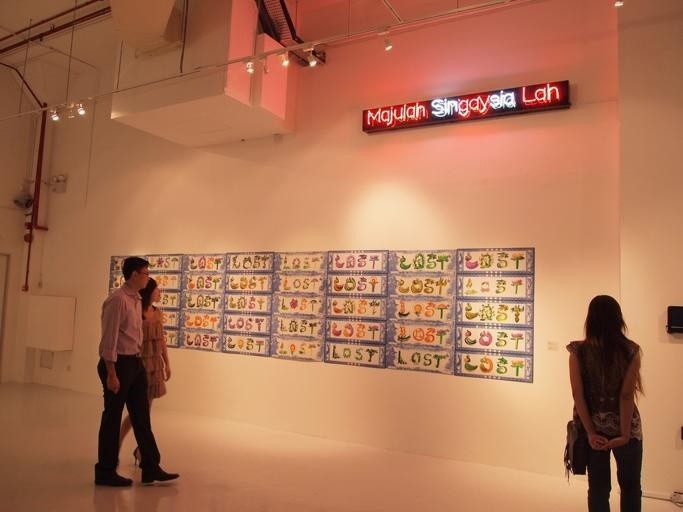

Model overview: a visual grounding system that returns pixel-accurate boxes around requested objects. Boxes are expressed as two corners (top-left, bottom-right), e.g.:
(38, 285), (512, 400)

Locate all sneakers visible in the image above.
(141, 464), (180, 484)
(95, 471), (133, 487)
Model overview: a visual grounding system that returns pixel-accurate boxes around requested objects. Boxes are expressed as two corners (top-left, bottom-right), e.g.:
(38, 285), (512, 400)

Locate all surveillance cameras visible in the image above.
(14, 198), (25, 208)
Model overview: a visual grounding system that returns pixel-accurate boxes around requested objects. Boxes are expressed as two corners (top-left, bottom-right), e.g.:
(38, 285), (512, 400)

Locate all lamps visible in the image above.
(0, 0), (624, 122)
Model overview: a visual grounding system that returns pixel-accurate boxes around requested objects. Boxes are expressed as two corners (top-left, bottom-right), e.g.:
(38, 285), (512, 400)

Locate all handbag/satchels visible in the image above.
(563, 419), (588, 476)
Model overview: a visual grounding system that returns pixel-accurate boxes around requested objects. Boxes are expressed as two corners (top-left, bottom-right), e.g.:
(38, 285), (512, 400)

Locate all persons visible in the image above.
(115, 276), (170, 470)
(566, 294), (644, 512)
(93, 256), (180, 487)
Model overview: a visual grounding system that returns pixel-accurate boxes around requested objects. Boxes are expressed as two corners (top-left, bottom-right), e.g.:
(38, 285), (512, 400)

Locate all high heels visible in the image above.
(132, 446), (143, 467)
